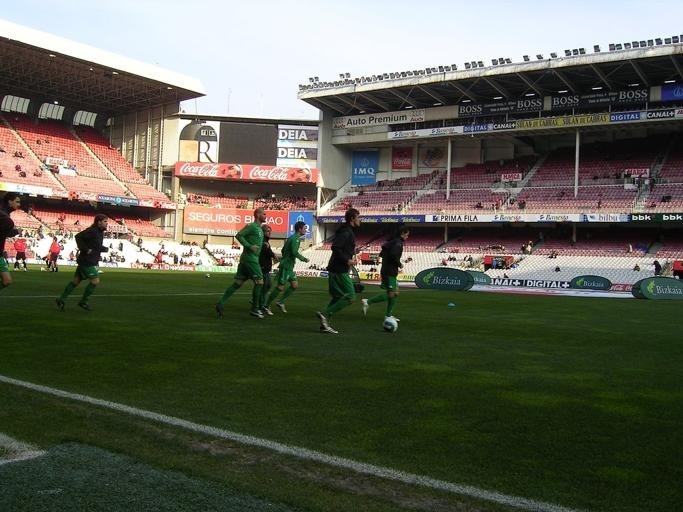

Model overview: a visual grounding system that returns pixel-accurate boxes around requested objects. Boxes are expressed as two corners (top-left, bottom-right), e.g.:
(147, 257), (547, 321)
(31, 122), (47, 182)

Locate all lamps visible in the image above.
(297, 29), (683, 96)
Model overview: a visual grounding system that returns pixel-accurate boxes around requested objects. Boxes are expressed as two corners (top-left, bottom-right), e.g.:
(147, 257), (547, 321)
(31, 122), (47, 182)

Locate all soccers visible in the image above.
(382, 318), (398, 333)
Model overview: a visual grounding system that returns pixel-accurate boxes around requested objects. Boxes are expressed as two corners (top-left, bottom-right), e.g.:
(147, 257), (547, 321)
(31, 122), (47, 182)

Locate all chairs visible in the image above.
(325, 135), (682, 215)
(319, 225), (680, 287)
(2, 207), (174, 268)
(176, 189), (315, 214)
(1, 117), (175, 205)
(184, 238), (318, 274)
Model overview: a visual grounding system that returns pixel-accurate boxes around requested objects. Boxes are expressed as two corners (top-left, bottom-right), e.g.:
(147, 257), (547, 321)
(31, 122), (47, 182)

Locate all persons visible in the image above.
(1, 145), (683, 272)
(56, 214), (113, 313)
(216, 209), (310, 319)
(316, 208), (415, 334)
(2, 192), (23, 288)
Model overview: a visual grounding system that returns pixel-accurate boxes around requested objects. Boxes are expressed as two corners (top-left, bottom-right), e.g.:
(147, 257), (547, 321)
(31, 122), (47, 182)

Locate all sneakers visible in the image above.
(54, 296), (65, 313)
(360, 298), (370, 320)
(384, 315), (400, 322)
(315, 310), (339, 334)
(248, 299), (288, 319)
(214, 301), (225, 320)
(77, 299), (93, 311)
